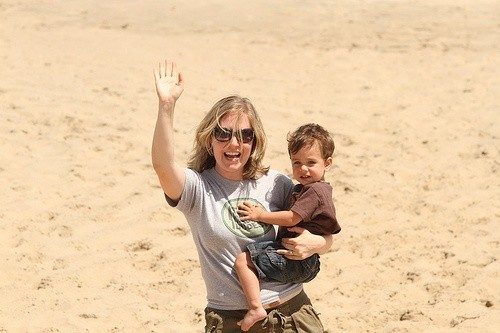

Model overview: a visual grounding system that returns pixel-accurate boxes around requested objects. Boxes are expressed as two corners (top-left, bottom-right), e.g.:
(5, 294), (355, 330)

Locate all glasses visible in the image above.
(213, 127), (254, 143)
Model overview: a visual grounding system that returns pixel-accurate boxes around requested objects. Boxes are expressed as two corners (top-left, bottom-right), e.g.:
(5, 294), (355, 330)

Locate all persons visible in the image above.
(233, 123), (341, 332)
(151, 57), (329, 333)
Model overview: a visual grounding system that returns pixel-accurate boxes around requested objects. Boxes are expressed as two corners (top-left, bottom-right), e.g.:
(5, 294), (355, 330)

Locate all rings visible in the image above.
(290, 250), (294, 255)
